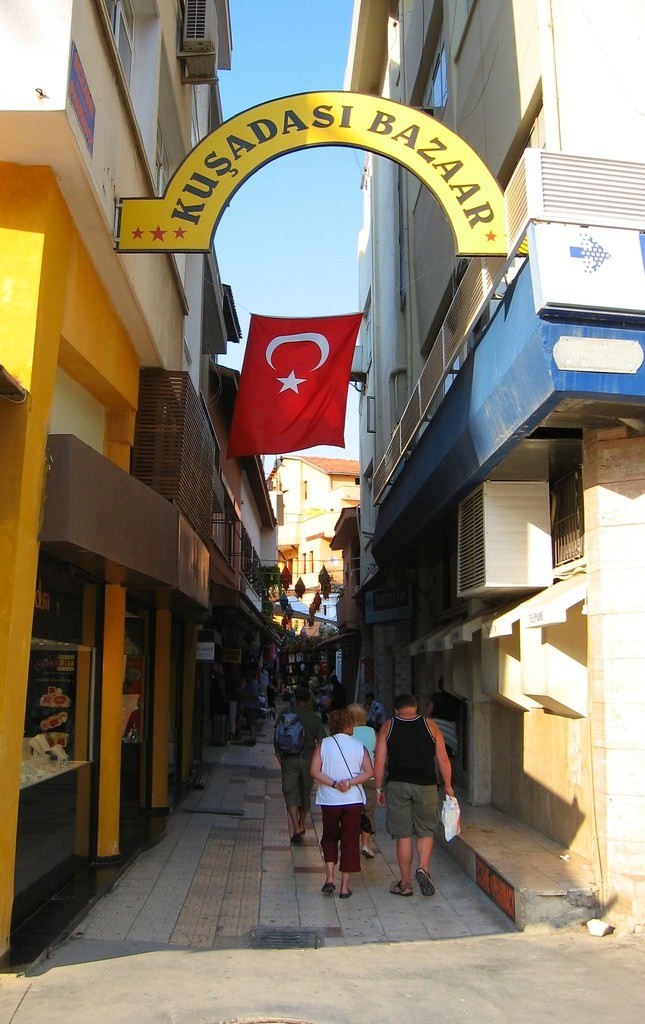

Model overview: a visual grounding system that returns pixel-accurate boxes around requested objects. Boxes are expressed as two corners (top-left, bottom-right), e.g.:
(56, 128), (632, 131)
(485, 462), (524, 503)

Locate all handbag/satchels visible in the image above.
(441, 794), (461, 841)
(361, 814), (372, 834)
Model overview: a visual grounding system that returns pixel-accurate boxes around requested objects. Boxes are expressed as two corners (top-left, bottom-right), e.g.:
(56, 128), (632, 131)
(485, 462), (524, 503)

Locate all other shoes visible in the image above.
(241, 739), (256, 747)
(362, 846), (375, 859)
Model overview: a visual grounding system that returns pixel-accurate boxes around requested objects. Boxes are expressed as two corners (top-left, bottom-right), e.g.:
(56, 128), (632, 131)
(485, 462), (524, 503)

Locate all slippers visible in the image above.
(339, 888), (352, 898)
(321, 882), (335, 893)
(298, 822), (305, 834)
(291, 831), (302, 842)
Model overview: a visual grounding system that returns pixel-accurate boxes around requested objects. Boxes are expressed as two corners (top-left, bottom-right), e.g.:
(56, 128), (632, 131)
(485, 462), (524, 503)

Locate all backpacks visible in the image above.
(274, 708), (311, 754)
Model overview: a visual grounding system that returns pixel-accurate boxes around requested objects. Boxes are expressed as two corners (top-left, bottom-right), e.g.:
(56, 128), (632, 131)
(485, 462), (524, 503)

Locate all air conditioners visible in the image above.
(456, 480), (553, 597)
(348, 346), (368, 381)
(177, 0), (218, 85)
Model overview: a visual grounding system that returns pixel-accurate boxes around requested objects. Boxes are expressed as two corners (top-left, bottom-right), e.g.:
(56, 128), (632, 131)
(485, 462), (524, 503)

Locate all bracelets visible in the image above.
(376, 788), (383, 793)
(346, 780), (351, 788)
(331, 781), (336, 788)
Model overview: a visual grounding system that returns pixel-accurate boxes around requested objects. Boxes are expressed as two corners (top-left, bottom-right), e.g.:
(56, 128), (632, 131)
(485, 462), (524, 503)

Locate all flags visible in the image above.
(227, 314), (361, 457)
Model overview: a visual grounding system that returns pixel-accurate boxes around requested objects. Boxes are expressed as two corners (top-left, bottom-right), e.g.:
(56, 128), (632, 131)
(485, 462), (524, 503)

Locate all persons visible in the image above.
(273, 689), (326, 843)
(373, 693), (454, 895)
(310, 708), (374, 898)
(345, 703), (377, 856)
(236, 672), (259, 745)
(363, 693), (386, 733)
(322, 676), (348, 723)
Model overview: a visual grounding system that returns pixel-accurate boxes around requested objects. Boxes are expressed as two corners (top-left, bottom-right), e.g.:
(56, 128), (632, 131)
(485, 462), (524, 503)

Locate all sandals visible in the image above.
(390, 880), (413, 896)
(414, 868), (436, 896)
